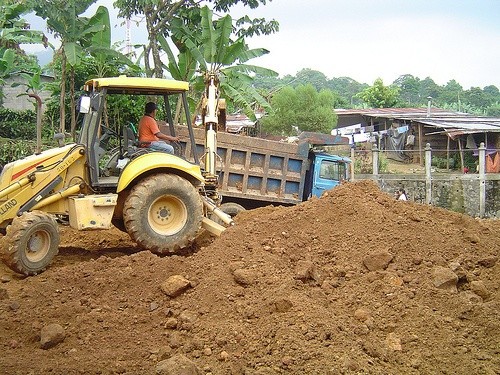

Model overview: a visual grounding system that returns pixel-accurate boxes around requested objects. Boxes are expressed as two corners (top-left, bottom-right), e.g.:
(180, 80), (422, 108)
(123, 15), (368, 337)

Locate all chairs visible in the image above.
(123, 120), (152, 160)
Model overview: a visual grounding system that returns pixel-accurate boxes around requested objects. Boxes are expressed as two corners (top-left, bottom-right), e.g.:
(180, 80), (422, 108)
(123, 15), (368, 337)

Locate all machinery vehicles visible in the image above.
(0, 74), (239, 277)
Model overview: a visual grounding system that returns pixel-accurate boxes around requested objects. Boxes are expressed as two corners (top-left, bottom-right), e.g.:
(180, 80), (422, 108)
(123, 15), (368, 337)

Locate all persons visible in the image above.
(397, 188), (407, 200)
(138, 101), (178, 154)
(394, 191), (401, 200)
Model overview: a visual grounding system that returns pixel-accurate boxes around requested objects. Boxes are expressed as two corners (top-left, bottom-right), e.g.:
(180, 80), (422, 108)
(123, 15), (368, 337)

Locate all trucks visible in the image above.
(158, 121), (353, 225)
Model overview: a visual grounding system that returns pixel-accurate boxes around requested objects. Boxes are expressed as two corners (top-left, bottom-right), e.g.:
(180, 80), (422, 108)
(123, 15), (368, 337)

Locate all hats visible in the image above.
(145, 101), (157, 113)
